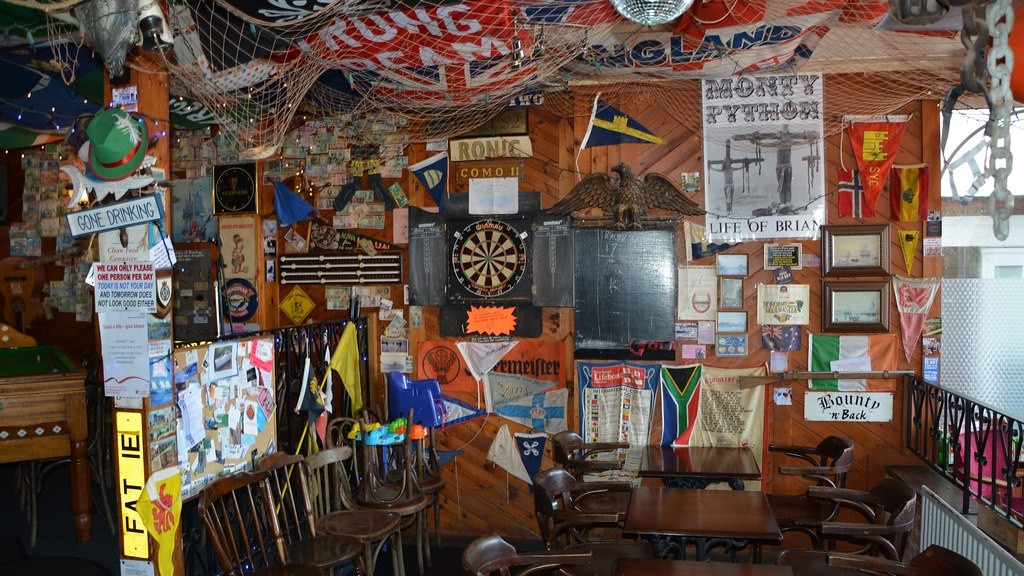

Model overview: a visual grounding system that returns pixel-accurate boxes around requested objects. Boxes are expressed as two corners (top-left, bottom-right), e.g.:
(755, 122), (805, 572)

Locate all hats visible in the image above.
(85, 106), (145, 184)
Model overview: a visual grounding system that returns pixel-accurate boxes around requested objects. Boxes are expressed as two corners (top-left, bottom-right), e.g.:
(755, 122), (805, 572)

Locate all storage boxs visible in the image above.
(975, 506), (1024, 554)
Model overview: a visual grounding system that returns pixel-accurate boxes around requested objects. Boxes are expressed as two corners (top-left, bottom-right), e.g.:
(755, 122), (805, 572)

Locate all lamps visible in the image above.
(136, 0), (184, 78)
(512, 18), (595, 65)
(609, 0), (695, 25)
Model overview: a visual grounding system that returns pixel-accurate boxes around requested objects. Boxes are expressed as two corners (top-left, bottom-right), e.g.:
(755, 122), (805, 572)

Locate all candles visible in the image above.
(716, 309), (749, 335)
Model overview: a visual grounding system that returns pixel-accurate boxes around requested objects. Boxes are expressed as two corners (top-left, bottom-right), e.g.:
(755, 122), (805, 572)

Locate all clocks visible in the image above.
(212, 162), (258, 216)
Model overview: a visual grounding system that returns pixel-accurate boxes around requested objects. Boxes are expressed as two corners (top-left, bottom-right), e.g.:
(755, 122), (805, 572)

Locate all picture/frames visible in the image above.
(715, 252), (750, 278)
(455, 107), (529, 140)
(720, 275), (745, 309)
(820, 224), (891, 278)
(821, 280), (891, 334)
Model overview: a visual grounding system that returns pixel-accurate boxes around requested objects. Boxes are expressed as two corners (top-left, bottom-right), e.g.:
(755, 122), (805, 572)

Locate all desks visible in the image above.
(0, 361), (92, 545)
(621, 486), (784, 564)
(638, 445), (762, 491)
(610, 557), (794, 576)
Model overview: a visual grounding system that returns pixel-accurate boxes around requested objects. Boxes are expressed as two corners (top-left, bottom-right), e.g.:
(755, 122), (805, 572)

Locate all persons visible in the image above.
(710, 159), (746, 213)
(747, 131), (812, 207)
(232, 234), (244, 274)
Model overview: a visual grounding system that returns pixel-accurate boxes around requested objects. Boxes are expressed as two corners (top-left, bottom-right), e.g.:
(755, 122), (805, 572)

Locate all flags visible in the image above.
(660, 363), (704, 445)
(888, 163), (929, 222)
(837, 166), (876, 219)
(328, 321), (362, 420)
(761, 323), (806, 349)
(661, 447), (694, 474)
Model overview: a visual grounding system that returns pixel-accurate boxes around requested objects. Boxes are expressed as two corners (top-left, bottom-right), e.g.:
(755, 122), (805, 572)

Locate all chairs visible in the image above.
(753, 436), (854, 564)
(23, 379), (116, 547)
(552, 429), (633, 544)
(827, 544), (983, 576)
(197, 469), (326, 576)
(13, 347), (100, 512)
(326, 417), (427, 576)
(532, 468), (654, 576)
(354, 407), (446, 567)
(775, 477), (917, 576)
(254, 451), (363, 576)
(461, 531), (593, 576)
(302, 446), (405, 576)
(948, 424), (1024, 526)
